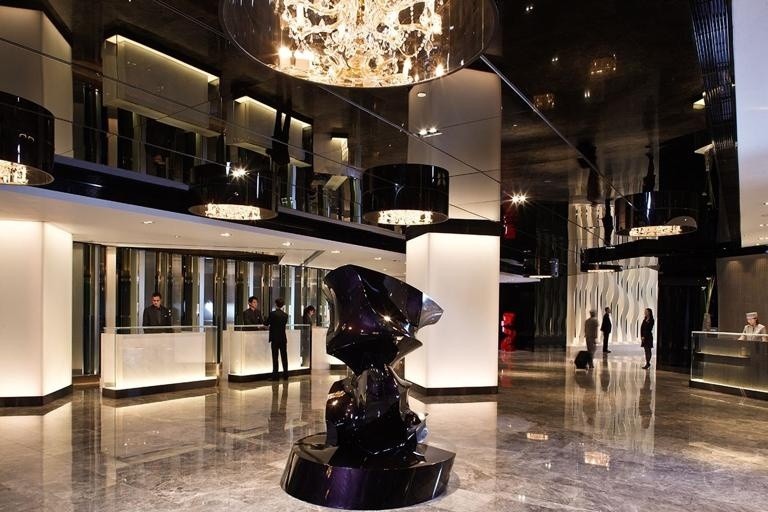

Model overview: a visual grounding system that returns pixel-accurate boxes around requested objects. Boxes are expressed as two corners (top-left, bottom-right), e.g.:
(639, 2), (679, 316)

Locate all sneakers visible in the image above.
(268, 373), (289, 382)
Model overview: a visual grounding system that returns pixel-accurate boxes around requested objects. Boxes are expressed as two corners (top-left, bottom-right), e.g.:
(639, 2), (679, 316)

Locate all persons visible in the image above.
(143, 292), (173, 333)
(300, 305), (315, 367)
(266, 87), (292, 172)
(642, 146), (656, 193)
(582, 368), (596, 424)
(600, 307), (612, 353)
(600, 352), (610, 391)
(640, 308), (654, 369)
(584, 310), (599, 368)
(268, 379), (288, 453)
(601, 198), (614, 246)
(263, 298), (289, 381)
(243, 296), (263, 331)
(300, 369), (310, 422)
(738, 311), (768, 342)
(638, 368), (652, 429)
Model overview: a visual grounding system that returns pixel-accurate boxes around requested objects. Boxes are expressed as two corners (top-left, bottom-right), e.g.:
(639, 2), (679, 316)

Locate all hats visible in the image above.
(745, 312), (758, 320)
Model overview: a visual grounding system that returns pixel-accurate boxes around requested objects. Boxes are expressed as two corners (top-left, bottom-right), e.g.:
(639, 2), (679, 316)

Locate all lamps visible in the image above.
(616, 135), (698, 236)
(360, 163), (449, 227)
(187, 161), (278, 225)
(523, 257), (560, 279)
(0, 91), (56, 186)
(218, 0), (501, 90)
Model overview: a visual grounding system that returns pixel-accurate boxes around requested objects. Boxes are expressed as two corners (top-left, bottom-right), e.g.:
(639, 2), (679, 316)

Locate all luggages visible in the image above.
(575, 350), (592, 371)
